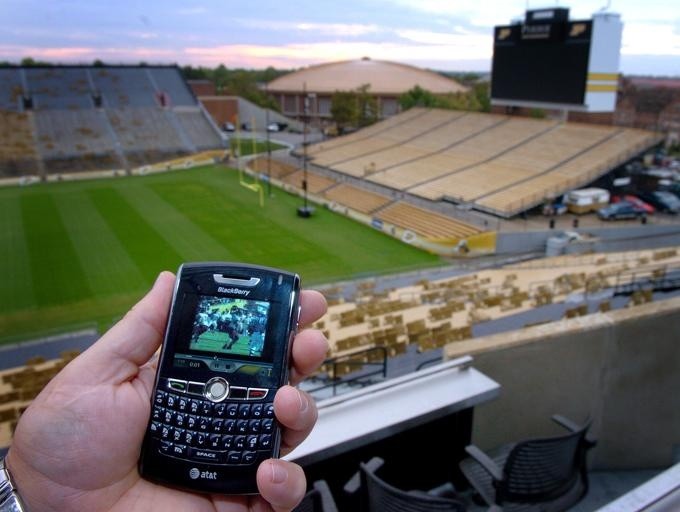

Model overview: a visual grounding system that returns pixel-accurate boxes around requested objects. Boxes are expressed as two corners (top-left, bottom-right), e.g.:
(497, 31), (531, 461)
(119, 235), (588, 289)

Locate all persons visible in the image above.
(194, 299), (267, 356)
(0, 266), (332, 512)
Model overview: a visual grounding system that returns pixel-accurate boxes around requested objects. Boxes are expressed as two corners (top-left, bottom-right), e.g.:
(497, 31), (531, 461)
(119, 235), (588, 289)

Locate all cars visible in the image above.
(562, 157), (679, 224)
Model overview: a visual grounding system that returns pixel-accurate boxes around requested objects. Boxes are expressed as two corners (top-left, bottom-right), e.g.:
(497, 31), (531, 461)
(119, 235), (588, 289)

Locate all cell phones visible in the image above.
(138, 262), (302, 496)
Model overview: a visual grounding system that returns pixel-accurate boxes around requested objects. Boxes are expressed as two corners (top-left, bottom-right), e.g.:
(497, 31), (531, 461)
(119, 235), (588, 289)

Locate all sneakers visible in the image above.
(191, 335), (263, 356)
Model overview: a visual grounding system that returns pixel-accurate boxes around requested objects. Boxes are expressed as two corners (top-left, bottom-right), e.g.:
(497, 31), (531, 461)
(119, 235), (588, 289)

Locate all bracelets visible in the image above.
(0, 460), (26, 511)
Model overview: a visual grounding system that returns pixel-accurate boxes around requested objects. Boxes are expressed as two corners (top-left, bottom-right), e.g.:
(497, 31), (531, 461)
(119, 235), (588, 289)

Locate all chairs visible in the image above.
(0, 246), (679, 459)
(299, 415), (597, 512)
